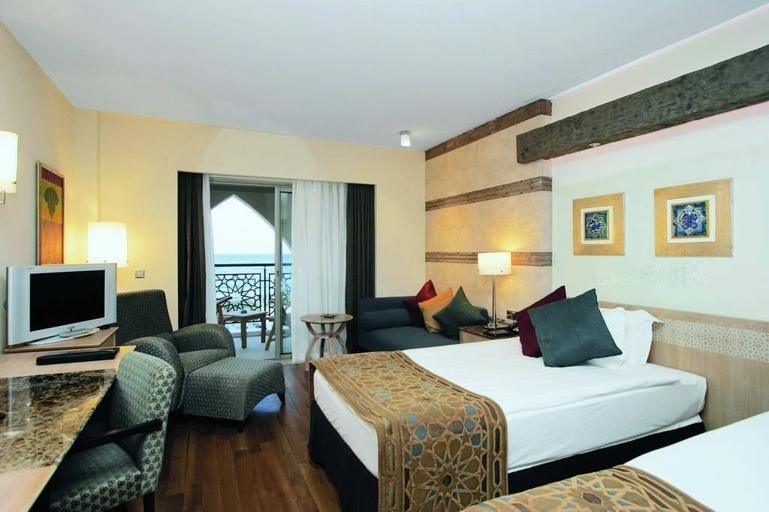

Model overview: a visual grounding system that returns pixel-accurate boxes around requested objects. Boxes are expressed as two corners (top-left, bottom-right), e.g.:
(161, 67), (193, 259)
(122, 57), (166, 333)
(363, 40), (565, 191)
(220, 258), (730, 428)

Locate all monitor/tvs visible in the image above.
(6, 262), (117, 346)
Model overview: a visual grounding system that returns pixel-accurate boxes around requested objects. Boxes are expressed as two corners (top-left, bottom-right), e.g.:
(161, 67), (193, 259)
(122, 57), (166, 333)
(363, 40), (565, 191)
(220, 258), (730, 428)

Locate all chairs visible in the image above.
(31, 352), (176, 512)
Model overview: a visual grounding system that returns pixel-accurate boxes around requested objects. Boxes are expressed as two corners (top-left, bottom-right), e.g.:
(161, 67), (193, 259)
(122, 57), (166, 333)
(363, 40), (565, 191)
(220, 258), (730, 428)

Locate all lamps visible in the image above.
(0, 129), (18, 206)
(400, 130), (411, 147)
(477, 252), (512, 329)
(87, 220), (126, 266)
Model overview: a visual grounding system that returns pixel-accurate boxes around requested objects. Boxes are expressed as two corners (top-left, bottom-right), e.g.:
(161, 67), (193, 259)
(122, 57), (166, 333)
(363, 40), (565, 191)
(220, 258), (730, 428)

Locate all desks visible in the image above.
(3, 325), (119, 351)
(1, 345), (137, 512)
(221, 311), (268, 349)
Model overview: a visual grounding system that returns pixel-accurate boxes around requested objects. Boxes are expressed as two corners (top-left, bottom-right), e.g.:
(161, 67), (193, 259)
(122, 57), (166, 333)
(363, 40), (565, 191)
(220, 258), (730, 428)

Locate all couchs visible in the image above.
(356, 295), (460, 349)
(115, 289), (235, 412)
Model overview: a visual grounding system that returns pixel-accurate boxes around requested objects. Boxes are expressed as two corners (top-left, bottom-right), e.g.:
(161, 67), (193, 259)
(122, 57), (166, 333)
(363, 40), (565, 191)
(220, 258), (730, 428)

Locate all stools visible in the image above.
(215, 295), (232, 327)
(182, 356), (286, 421)
(265, 307), (290, 354)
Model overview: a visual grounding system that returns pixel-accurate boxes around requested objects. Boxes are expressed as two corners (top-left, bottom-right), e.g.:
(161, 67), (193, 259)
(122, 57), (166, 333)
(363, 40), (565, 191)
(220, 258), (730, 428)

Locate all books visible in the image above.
(36, 346), (120, 364)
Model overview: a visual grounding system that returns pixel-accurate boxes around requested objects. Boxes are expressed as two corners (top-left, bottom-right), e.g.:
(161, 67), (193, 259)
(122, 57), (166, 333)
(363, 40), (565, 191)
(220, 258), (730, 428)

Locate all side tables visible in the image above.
(301, 313), (353, 372)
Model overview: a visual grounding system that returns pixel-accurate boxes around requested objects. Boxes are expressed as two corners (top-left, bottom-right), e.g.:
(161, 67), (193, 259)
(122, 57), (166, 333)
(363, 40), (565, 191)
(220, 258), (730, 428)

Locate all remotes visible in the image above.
(37, 348), (120, 366)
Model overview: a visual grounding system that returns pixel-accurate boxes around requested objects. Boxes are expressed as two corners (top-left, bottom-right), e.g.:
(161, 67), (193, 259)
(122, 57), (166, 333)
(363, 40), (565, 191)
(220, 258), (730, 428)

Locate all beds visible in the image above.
(458, 412), (769, 512)
(308, 332), (708, 511)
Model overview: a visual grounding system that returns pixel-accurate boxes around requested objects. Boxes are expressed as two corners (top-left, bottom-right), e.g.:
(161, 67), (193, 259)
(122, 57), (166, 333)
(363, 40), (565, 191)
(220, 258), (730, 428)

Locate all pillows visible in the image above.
(528, 289), (623, 367)
(584, 306), (664, 370)
(434, 286), (489, 340)
(419, 289), (454, 333)
(514, 287), (564, 357)
(405, 280), (442, 328)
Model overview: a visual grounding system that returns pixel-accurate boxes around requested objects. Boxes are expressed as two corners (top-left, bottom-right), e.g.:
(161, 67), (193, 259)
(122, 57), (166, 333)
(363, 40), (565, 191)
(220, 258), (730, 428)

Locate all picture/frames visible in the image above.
(37, 162), (66, 266)
(655, 178), (732, 258)
(573, 194), (625, 256)
